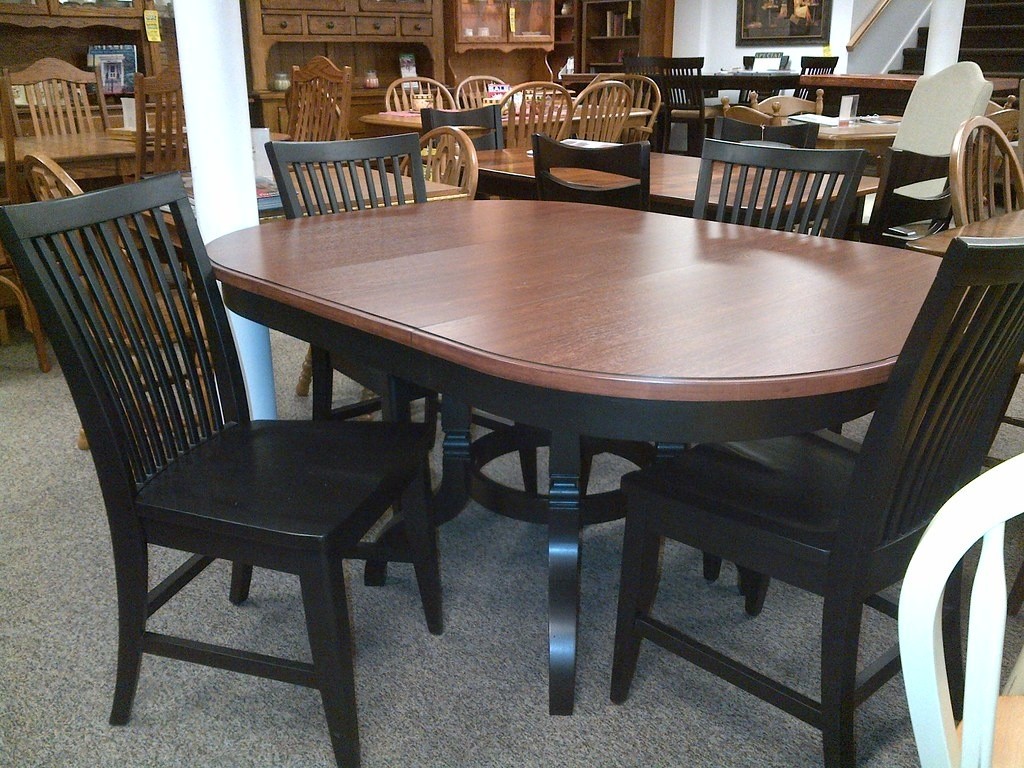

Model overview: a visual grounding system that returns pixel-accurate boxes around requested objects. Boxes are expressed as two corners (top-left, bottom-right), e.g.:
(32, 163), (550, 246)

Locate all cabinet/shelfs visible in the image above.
(582, 0), (674, 75)
(547, 0), (582, 86)
(1, 0), (188, 138)
(245, 0), (446, 170)
(443, 0), (556, 53)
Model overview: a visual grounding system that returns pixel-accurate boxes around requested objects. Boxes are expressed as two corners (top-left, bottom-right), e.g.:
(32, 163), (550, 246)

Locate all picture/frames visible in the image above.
(734, 0), (831, 47)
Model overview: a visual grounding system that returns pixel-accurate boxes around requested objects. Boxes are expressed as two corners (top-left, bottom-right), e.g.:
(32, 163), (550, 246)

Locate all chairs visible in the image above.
(0, 171), (444, 766)
(0, 53), (1023, 421)
(612, 235), (1024, 768)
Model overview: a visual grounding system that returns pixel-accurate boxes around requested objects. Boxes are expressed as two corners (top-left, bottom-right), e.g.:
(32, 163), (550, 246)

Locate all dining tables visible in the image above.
(619, 71), (798, 157)
(418, 145), (882, 230)
(779, 115), (903, 175)
(202, 199), (990, 715)
(1, 125), (293, 209)
(126, 163), (469, 253)
(357, 102), (652, 158)
